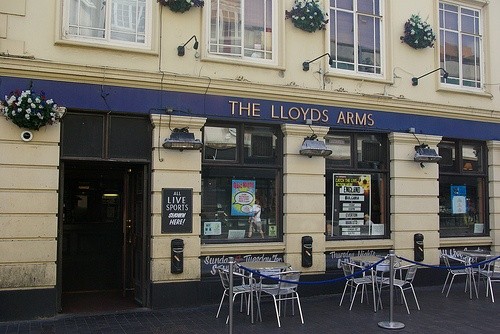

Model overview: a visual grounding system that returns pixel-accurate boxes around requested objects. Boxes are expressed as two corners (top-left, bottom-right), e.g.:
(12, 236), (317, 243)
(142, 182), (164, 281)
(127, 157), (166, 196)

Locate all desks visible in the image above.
(352, 256), (401, 303)
(457, 251), (495, 294)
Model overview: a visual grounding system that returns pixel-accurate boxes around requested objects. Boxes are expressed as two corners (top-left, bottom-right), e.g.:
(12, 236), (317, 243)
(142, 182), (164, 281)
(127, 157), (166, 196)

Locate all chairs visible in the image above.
(441, 247), (500, 303)
(340, 255), (420, 314)
(215, 261), (305, 327)
(237, 261), (293, 322)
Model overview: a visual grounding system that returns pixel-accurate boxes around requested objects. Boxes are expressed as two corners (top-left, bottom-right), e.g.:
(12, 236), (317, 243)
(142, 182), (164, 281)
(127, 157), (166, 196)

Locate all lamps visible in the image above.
(177, 35), (198, 56)
(412, 68), (449, 86)
(162, 128), (204, 152)
(299, 134), (333, 158)
(303, 53), (333, 71)
(414, 145), (443, 168)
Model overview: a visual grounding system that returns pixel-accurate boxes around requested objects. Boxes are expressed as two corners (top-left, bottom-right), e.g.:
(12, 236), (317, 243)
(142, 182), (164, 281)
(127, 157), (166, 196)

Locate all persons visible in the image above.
(247, 199), (264, 238)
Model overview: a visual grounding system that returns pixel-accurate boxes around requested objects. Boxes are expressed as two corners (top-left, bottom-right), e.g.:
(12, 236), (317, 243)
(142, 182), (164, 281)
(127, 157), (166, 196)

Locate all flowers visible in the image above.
(0, 90), (67, 129)
(285, 0), (329, 33)
(400, 13), (436, 50)
(155, 0), (205, 14)
